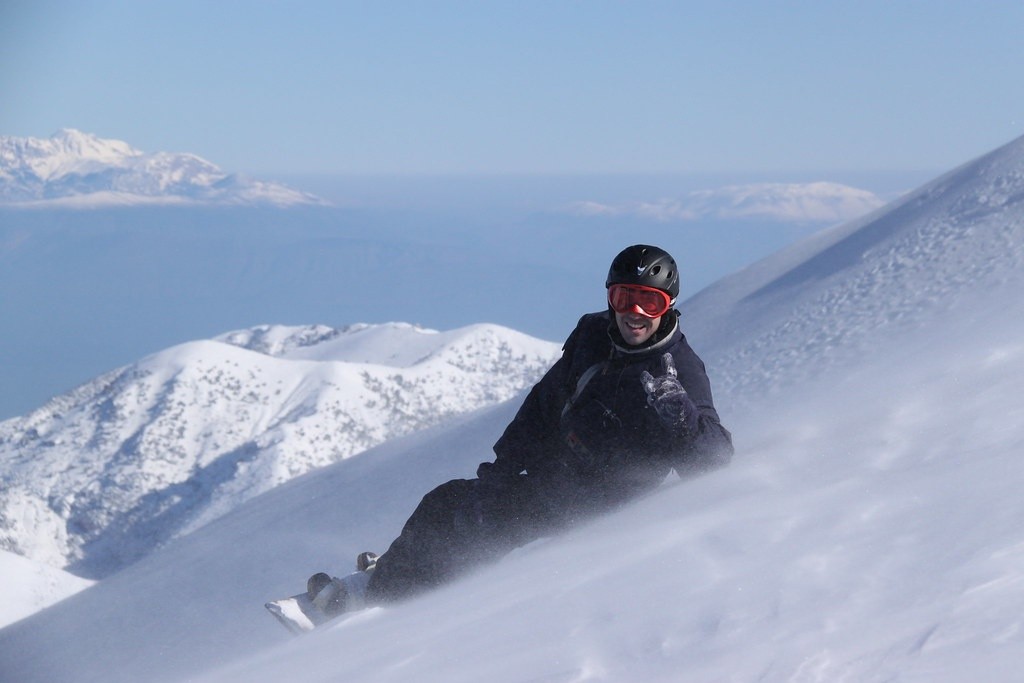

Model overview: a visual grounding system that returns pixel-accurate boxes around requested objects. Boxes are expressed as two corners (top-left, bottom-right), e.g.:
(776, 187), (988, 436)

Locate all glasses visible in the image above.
(606, 284), (677, 318)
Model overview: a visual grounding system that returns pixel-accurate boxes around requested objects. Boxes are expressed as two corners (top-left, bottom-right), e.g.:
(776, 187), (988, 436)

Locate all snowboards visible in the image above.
(263, 568), (380, 639)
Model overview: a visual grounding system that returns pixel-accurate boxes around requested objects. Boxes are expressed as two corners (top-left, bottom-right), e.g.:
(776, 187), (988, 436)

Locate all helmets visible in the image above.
(605, 244), (680, 308)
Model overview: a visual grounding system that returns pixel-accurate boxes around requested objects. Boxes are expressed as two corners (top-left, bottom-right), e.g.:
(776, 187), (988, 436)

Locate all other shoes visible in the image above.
(356, 551), (380, 573)
(306, 572), (349, 617)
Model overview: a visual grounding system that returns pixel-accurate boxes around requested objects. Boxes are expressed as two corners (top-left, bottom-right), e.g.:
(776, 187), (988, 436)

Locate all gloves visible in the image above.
(639, 352), (693, 433)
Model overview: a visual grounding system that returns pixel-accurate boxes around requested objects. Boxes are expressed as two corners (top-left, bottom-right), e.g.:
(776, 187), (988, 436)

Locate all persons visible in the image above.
(307, 245), (735, 619)
